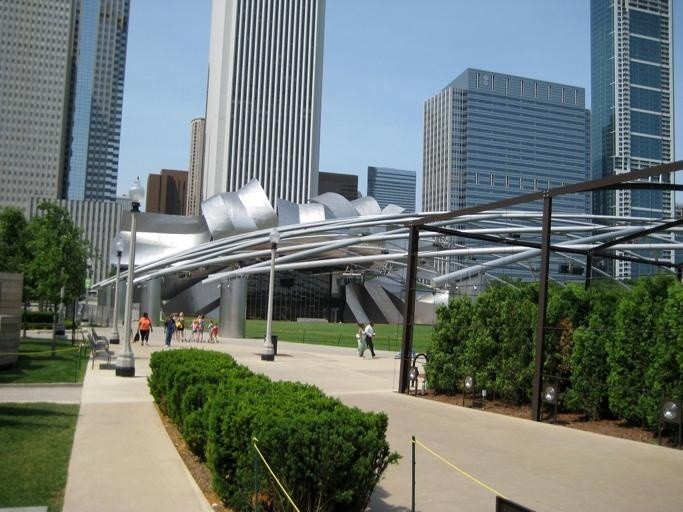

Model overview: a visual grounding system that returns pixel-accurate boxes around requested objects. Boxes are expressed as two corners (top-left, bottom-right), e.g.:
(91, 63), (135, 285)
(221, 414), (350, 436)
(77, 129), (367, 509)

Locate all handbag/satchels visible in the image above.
(176, 322), (183, 329)
(133, 333), (139, 342)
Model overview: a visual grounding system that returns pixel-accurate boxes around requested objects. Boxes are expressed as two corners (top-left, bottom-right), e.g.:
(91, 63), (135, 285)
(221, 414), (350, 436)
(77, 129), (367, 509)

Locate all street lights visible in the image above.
(108, 236), (125, 344)
(114, 174), (146, 378)
(260, 223), (282, 361)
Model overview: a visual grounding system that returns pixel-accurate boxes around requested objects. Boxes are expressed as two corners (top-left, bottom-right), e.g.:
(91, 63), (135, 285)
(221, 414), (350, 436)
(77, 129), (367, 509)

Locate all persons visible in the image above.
(136, 312), (153, 345)
(356, 321), (366, 357)
(171, 312), (219, 344)
(162, 313), (175, 347)
(364, 320), (375, 358)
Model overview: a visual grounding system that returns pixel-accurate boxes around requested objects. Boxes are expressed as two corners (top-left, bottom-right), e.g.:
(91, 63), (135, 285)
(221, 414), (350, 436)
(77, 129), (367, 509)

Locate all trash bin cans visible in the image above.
(264, 334), (278, 354)
(55, 326), (65, 338)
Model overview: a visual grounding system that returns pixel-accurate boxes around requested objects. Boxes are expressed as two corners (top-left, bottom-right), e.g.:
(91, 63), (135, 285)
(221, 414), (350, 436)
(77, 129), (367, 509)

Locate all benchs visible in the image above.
(87, 326), (112, 369)
